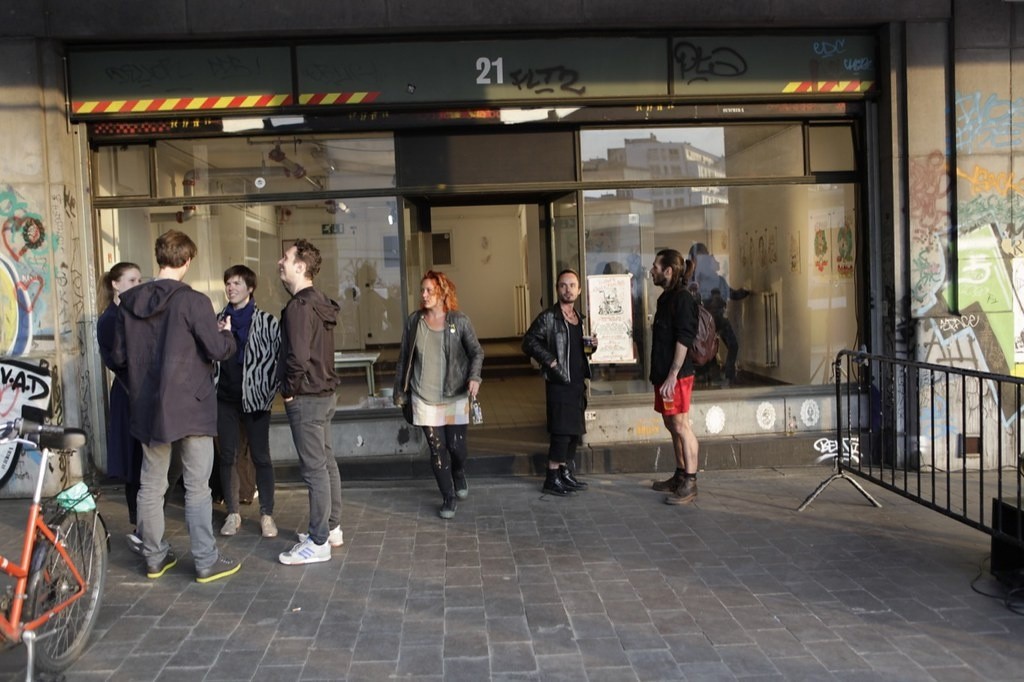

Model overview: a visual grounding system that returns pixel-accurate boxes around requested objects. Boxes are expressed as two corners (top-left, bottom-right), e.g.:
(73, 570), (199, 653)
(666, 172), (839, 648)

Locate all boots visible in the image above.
(664, 472), (698, 504)
(559, 462), (589, 490)
(542, 464), (576, 496)
(652, 467), (685, 492)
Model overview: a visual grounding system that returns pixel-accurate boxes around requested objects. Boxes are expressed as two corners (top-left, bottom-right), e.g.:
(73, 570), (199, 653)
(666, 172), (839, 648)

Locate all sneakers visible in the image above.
(261, 515), (278, 538)
(196, 556), (240, 582)
(221, 513), (242, 535)
(147, 550), (177, 577)
(279, 534), (331, 564)
(299, 525), (343, 547)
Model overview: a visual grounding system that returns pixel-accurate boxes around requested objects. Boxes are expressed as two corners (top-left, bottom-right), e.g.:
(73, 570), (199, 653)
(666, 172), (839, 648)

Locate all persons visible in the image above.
(393, 271), (485, 518)
(212, 264), (283, 538)
(98, 263), (142, 554)
(648, 249), (718, 505)
(521, 270), (598, 497)
(602, 242), (755, 388)
(112, 230), (242, 582)
(277, 239), (344, 566)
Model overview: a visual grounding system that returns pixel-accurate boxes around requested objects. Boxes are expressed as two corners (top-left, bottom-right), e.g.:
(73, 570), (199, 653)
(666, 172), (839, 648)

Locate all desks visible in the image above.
(333, 353), (381, 396)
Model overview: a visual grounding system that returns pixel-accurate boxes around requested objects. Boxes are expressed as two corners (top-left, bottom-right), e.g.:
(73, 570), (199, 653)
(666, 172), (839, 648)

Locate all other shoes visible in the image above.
(440, 503), (457, 518)
(126, 533), (143, 554)
(453, 476), (469, 501)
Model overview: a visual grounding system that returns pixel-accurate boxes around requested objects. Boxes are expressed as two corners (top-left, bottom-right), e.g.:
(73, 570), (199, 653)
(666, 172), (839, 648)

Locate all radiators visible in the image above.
(737, 291), (778, 369)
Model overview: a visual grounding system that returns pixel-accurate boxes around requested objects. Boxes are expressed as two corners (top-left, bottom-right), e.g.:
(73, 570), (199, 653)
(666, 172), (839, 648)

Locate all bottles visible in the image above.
(470, 396), (483, 425)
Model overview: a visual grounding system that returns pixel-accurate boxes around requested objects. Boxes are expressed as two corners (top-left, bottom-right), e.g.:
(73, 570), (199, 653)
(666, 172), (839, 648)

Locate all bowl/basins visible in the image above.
(379, 387), (393, 396)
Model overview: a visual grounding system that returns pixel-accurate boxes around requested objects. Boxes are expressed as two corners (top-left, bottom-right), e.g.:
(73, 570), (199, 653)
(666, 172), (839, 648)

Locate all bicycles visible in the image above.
(0, 419), (113, 681)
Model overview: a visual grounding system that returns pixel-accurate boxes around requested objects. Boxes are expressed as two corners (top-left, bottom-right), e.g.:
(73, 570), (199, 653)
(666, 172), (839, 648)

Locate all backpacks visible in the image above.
(681, 288), (720, 365)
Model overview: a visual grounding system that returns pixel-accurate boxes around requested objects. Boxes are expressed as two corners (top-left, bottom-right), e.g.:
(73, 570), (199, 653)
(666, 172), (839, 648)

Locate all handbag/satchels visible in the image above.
(402, 391), (413, 424)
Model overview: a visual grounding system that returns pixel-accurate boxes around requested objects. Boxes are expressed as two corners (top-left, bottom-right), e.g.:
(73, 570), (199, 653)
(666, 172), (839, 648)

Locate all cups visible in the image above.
(583, 336), (593, 353)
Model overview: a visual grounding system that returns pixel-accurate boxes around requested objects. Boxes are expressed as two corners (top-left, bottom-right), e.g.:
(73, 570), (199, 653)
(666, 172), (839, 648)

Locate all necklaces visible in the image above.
(562, 310), (576, 320)
